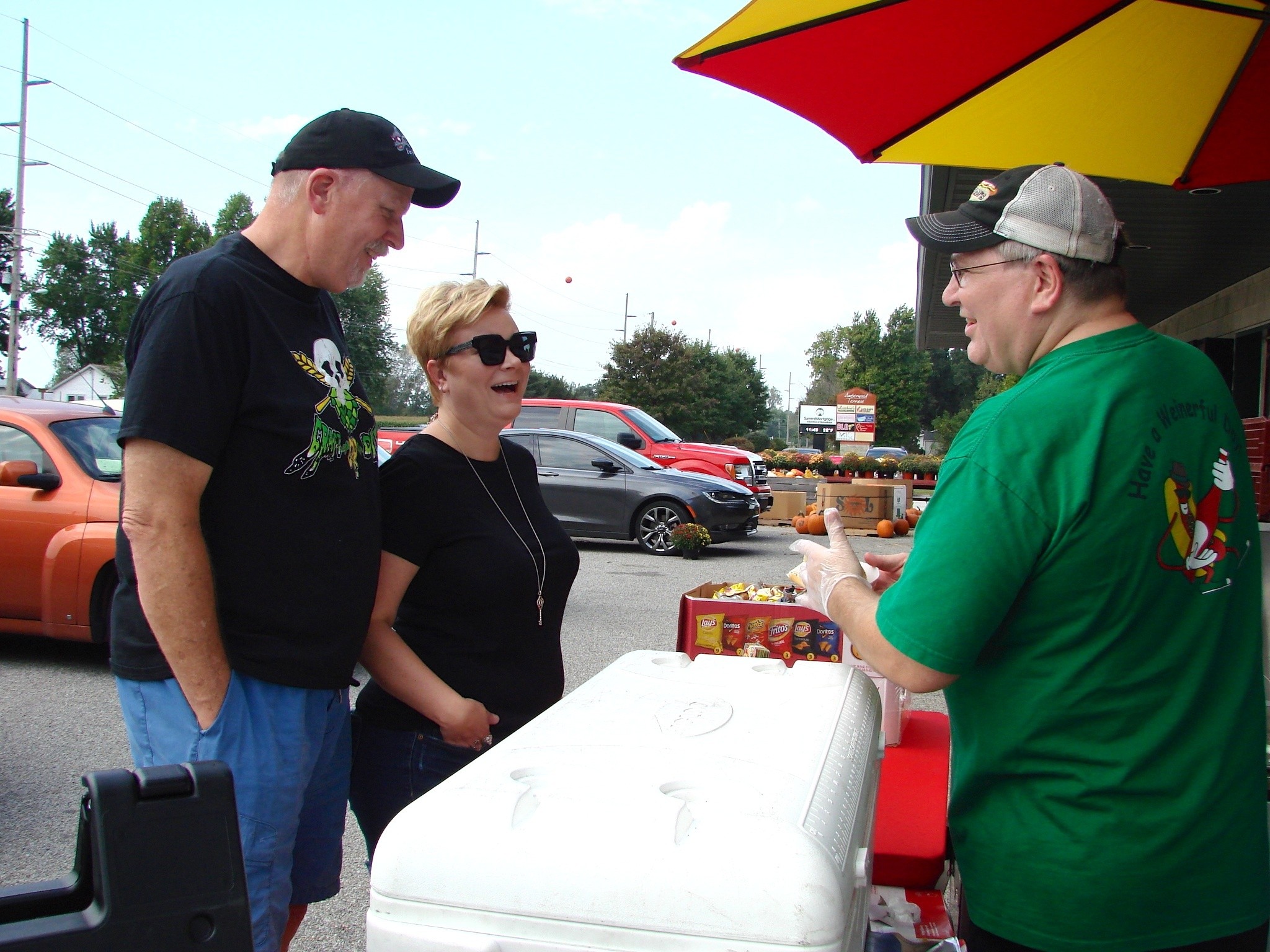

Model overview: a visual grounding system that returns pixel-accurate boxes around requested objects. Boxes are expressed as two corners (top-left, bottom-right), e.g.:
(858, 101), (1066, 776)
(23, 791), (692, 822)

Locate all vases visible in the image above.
(682, 547), (700, 560)
(768, 465), (937, 481)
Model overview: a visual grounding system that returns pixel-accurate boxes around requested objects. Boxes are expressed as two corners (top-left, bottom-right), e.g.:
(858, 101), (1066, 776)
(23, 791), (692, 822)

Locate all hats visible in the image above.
(269, 108), (461, 209)
(904, 162), (1124, 264)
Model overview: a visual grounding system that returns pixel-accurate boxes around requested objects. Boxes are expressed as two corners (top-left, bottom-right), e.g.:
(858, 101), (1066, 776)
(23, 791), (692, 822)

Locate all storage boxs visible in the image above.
(843, 632), (912, 748)
(759, 478), (913, 529)
(367, 650), (885, 952)
(676, 581), (843, 667)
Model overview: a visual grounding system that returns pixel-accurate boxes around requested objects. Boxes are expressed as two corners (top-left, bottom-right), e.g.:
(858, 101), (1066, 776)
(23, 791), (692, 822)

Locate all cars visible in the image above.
(782, 448), (822, 455)
(0, 395), (125, 664)
(500, 429), (760, 557)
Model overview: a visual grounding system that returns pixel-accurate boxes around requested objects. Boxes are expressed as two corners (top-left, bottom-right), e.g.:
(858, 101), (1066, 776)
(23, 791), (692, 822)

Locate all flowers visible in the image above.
(669, 523), (712, 550)
(755, 448), (941, 475)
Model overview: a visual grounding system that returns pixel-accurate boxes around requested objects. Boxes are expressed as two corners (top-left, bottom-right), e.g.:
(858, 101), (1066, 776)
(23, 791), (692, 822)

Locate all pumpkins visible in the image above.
(877, 519), (894, 538)
(905, 506), (922, 528)
(769, 466), (824, 478)
(894, 514), (909, 535)
(792, 503), (827, 535)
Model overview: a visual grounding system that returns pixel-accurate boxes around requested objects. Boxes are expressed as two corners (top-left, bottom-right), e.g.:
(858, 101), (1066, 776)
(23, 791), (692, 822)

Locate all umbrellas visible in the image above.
(671, 0), (1270, 189)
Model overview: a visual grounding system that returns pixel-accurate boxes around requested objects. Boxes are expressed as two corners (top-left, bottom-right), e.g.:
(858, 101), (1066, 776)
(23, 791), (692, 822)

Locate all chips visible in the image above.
(699, 635), (831, 652)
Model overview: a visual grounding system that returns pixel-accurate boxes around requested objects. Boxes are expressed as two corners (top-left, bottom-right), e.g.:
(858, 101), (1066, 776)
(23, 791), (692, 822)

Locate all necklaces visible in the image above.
(436, 416), (545, 625)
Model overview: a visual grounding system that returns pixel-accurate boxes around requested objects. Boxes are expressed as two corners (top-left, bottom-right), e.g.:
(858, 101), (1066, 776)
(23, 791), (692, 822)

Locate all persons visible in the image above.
(108, 108), (461, 952)
(789, 161), (1270, 952)
(351, 279), (579, 878)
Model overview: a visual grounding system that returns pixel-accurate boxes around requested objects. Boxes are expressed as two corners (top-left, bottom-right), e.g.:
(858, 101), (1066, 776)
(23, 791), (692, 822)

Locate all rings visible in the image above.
(472, 731), (492, 751)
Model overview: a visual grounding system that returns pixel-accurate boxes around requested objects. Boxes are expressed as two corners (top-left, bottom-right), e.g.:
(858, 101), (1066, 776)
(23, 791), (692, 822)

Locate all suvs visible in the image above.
(866, 445), (908, 459)
(501, 398), (774, 513)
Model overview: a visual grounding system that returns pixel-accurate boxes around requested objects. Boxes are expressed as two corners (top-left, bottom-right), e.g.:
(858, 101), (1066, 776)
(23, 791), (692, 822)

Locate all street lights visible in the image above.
(6, 289), (48, 396)
(777, 419), (781, 438)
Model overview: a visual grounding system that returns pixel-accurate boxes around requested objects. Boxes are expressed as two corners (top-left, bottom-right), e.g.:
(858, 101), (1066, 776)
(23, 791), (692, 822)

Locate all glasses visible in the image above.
(948, 255), (1028, 288)
(437, 330), (539, 367)
